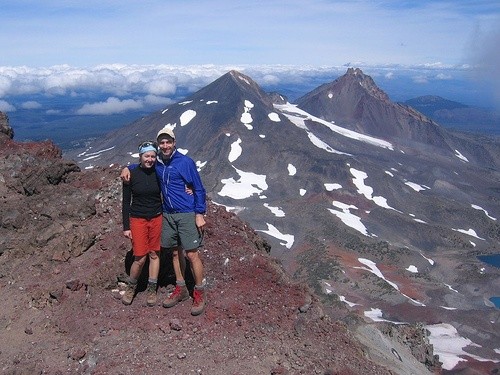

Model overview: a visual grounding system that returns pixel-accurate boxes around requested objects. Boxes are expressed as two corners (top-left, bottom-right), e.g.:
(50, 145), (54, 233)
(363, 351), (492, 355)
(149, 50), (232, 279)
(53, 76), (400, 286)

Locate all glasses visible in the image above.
(143, 143), (156, 147)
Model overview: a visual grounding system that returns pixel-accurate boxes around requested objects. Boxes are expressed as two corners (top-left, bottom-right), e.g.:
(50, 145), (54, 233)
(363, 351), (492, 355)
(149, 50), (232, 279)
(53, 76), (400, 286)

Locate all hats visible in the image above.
(138, 140), (158, 156)
(156, 128), (176, 143)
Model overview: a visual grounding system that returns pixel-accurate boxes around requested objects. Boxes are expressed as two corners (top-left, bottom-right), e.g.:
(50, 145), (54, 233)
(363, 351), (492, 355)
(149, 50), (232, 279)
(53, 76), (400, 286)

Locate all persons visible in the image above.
(122, 141), (194, 307)
(121, 128), (207, 316)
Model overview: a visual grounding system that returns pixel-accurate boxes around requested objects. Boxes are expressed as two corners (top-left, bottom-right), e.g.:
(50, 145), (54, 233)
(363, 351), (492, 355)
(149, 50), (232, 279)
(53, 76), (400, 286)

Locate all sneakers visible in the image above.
(146, 280), (158, 305)
(121, 282), (137, 305)
(191, 285), (207, 315)
(162, 280), (190, 308)
